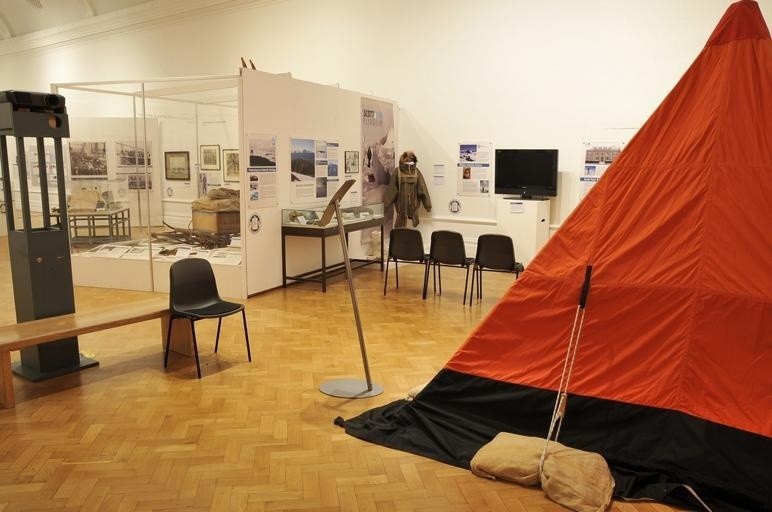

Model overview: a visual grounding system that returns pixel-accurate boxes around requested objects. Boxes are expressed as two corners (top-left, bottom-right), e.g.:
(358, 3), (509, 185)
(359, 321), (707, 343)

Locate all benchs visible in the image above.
(0, 296), (197, 411)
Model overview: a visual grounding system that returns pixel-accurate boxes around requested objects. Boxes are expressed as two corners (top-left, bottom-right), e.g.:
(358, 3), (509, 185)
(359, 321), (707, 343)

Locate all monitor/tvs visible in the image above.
(494, 148), (558, 201)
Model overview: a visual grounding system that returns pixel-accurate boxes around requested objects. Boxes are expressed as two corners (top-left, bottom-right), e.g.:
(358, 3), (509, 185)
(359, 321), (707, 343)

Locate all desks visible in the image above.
(281, 206), (384, 293)
(495, 198), (550, 270)
(50, 206), (131, 246)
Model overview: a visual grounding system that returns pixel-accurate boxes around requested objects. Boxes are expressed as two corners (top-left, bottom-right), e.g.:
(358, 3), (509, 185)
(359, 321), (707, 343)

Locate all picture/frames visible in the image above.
(165, 151), (190, 181)
(345, 151), (359, 173)
(223, 149), (240, 182)
(200, 145), (220, 170)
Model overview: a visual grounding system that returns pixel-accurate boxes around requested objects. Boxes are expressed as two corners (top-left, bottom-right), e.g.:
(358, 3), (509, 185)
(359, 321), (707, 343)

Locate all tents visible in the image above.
(332, 1), (772, 512)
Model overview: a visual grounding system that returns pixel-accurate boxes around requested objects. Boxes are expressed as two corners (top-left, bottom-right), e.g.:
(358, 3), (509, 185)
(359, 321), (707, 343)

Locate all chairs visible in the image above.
(164, 257), (252, 379)
(383, 228), (437, 300)
(425, 230), (479, 306)
(470, 234), (523, 306)
